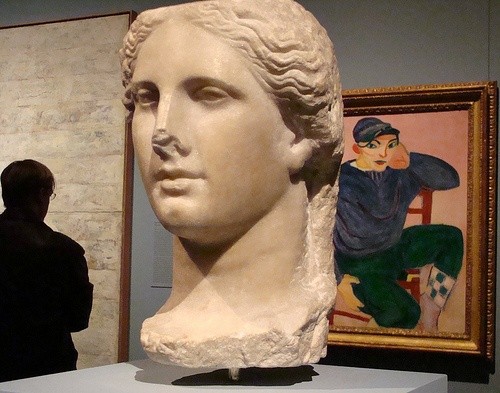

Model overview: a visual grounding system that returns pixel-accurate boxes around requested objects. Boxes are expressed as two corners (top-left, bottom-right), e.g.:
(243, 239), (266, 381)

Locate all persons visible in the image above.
(118, 0), (346, 371)
(0, 159), (94, 385)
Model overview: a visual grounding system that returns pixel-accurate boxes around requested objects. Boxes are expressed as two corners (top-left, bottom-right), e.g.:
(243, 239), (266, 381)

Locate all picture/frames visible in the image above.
(0, 10), (139, 364)
(325, 81), (499, 365)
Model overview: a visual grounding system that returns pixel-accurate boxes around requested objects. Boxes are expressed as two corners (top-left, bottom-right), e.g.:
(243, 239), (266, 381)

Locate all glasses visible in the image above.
(19, 183), (57, 199)
